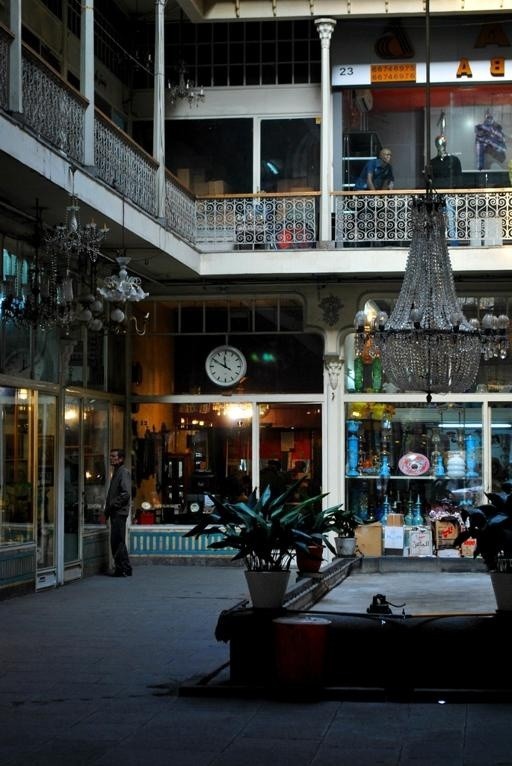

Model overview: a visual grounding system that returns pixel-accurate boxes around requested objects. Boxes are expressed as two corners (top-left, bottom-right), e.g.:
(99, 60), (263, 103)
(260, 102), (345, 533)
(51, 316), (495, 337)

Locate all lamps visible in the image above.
(349, 0), (511, 405)
(33, 158), (111, 264)
(55, 189), (154, 337)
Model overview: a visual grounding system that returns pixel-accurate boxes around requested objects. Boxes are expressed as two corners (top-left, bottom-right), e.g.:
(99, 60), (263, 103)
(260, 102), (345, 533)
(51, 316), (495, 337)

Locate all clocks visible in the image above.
(205, 346), (248, 389)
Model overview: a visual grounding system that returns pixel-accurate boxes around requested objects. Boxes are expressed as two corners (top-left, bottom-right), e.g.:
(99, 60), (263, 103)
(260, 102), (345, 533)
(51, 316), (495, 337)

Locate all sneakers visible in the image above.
(110, 569), (132, 577)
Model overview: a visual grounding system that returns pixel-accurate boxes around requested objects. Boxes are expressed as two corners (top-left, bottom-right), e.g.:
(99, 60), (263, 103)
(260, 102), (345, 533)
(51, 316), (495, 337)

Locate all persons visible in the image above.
(426, 135), (464, 246)
(134, 462), (161, 522)
(101, 446), (134, 579)
(225, 460), (294, 504)
(348, 146), (396, 246)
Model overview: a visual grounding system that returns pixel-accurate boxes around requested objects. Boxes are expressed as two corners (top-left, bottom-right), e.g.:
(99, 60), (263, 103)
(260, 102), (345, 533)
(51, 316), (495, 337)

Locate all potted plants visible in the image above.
(171, 473), (365, 612)
